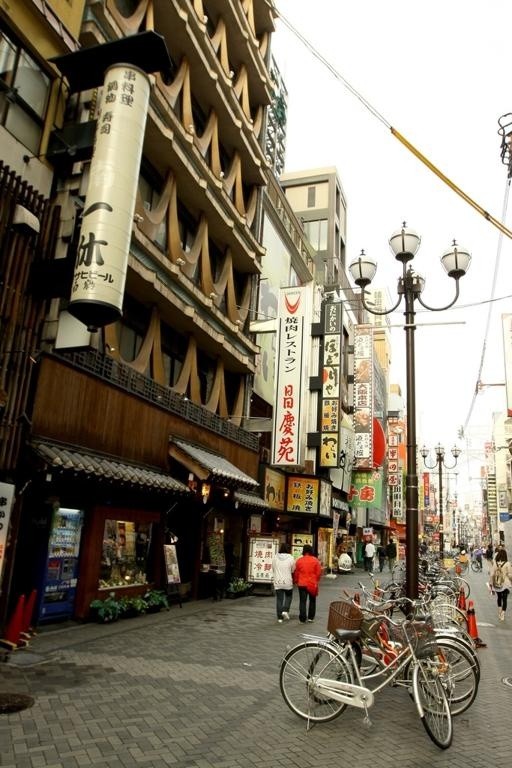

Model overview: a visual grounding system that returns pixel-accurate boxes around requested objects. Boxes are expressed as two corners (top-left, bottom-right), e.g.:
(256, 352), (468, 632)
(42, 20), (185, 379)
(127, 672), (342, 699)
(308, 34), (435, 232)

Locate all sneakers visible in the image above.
(298, 617), (314, 625)
(276, 611), (290, 624)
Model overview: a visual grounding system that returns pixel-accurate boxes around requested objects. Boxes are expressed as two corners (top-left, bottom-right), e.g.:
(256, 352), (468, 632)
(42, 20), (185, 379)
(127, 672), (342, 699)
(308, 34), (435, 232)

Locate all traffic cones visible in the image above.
(460, 587), (466, 610)
(466, 599), (482, 644)
(4, 589), (37, 645)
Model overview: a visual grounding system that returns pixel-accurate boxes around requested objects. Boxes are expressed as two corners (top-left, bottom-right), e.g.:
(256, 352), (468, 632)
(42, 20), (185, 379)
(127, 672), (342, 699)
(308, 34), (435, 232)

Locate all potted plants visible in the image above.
(88, 588), (171, 624)
(227, 575), (253, 599)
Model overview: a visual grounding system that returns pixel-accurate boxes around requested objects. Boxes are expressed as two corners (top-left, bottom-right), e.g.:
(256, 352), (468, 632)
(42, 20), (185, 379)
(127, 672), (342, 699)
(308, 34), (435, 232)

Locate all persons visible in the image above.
(293, 545), (322, 625)
(365, 540), (376, 572)
(457, 543), (512, 620)
(272, 543), (296, 623)
(376, 542), (387, 572)
(419, 542), (428, 557)
(386, 539), (396, 569)
(362, 541), (369, 571)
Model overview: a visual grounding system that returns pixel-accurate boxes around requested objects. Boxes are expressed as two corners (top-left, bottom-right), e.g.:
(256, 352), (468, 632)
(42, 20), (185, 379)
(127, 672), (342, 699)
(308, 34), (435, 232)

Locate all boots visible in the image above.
(497, 607), (506, 622)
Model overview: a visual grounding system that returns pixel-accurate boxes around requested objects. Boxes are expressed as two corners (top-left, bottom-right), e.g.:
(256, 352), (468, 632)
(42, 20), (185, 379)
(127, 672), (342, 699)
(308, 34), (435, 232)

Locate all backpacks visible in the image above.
(492, 560), (507, 589)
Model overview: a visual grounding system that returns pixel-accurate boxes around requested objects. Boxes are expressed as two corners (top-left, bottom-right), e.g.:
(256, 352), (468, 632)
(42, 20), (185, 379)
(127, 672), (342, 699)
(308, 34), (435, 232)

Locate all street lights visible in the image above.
(348, 221), (473, 617)
(420, 442), (461, 560)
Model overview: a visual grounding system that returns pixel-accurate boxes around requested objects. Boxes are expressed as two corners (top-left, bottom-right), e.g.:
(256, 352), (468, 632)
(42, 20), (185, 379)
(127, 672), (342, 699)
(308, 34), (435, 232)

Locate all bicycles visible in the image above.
(279, 553), (480, 749)
